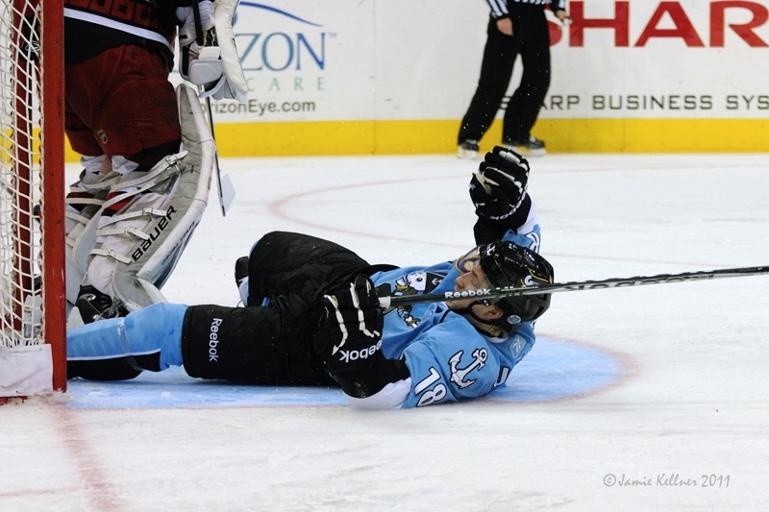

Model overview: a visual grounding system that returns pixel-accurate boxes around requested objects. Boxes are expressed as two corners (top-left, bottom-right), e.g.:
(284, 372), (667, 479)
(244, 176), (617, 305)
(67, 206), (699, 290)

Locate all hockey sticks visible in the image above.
(190, 1), (235, 216)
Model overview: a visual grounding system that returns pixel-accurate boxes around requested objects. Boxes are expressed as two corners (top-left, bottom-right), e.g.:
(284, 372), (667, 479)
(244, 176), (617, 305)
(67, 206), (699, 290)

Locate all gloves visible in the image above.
(316, 277), (383, 370)
(470, 152), (531, 232)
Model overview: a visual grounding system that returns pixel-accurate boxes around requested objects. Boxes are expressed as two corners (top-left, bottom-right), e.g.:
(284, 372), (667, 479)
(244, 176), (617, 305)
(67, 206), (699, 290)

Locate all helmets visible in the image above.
(477, 239), (554, 333)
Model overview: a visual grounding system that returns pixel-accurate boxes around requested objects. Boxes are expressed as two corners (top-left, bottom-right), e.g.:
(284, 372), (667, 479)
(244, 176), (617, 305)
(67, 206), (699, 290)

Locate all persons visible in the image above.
(65, 145), (555, 409)
(456, 1), (566, 159)
(0, 0), (251, 324)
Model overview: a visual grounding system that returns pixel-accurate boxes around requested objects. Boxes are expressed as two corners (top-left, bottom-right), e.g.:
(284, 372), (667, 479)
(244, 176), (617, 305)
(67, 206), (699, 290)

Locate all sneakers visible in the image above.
(460, 140), (479, 154)
(502, 135), (546, 152)
